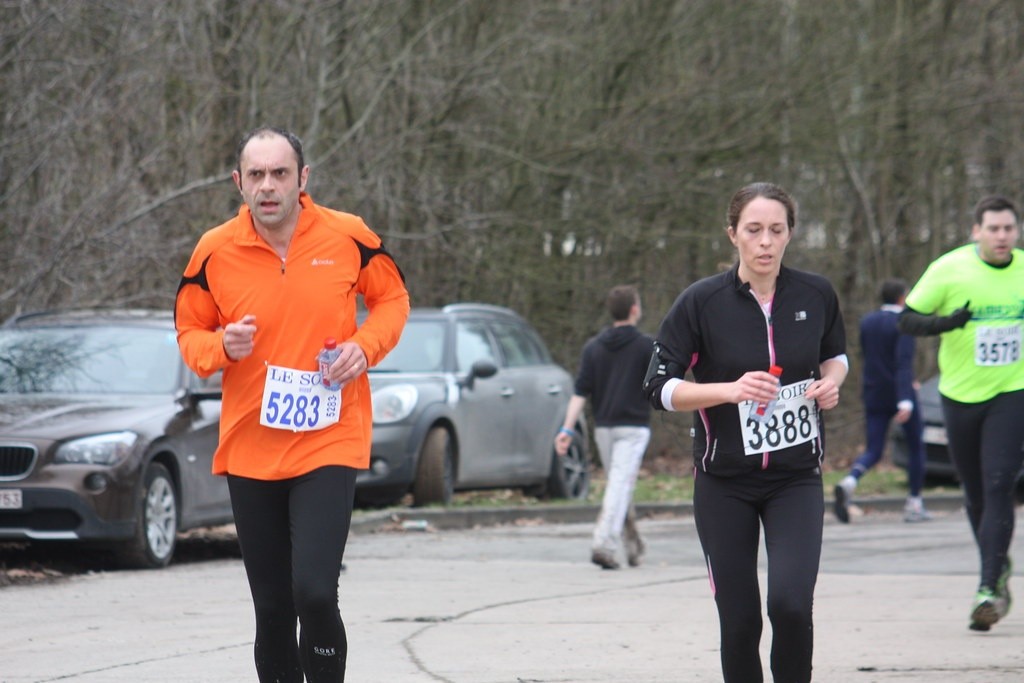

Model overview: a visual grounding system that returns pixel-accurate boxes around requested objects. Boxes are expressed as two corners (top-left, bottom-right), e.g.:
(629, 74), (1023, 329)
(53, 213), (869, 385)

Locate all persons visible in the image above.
(833, 280), (934, 523)
(174, 126), (410, 683)
(896, 195), (1023, 632)
(554, 285), (656, 570)
(642, 182), (849, 683)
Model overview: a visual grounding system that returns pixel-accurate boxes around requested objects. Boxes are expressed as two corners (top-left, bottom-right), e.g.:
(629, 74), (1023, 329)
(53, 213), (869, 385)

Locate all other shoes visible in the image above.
(904, 507), (933, 521)
(592, 549), (622, 569)
(621, 537), (645, 566)
(833, 481), (851, 523)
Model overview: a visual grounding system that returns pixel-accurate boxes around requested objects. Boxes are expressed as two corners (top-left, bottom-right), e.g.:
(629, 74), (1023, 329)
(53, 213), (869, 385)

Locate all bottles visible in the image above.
(749, 365), (783, 425)
(318, 339), (341, 391)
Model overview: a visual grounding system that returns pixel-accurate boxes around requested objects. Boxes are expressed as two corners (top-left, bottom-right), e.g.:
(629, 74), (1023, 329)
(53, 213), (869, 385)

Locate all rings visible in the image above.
(355, 364), (359, 370)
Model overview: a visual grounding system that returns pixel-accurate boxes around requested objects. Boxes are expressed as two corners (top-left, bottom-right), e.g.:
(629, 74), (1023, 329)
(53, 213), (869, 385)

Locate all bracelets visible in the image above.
(559, 429), (573, 437)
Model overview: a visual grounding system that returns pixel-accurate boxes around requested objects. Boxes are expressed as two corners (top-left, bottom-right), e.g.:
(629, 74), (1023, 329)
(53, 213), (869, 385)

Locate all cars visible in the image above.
(0, 310), (232, 570)
(353, 303), (591, 512)
(884, 370), (1023, 505)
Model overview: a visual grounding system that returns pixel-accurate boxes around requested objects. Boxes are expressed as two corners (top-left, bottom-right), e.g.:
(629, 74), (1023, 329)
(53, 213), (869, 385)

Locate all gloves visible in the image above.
(952, 300), (973, 327)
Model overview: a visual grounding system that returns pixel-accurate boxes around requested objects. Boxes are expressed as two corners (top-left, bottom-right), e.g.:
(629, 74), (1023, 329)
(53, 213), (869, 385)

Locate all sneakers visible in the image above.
(968, 586), (999, 631)
(996, 555), (1012, 615)
